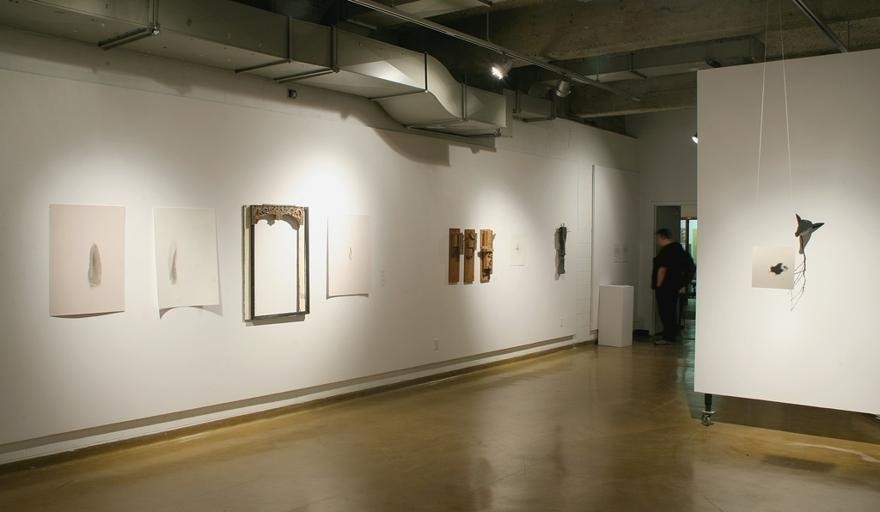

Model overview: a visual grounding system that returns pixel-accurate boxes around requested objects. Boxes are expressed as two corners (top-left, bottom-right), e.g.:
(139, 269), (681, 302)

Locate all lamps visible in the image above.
(489, 53), (512, 81)
(555, 78), (572, 99)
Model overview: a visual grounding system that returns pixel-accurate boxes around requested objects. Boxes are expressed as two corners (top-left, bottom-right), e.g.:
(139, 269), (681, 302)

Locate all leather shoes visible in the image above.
(656, 340), (680, 345)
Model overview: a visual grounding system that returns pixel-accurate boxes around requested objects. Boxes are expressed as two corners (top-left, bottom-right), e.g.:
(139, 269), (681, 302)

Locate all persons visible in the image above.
(652, 228), (684, 345)
